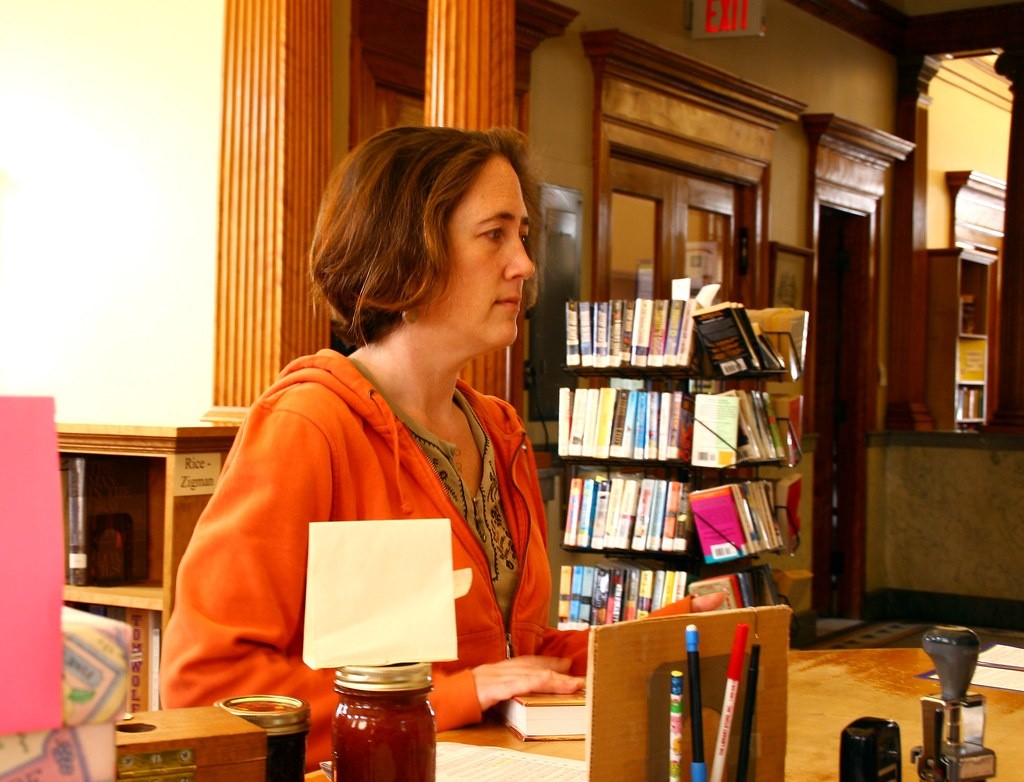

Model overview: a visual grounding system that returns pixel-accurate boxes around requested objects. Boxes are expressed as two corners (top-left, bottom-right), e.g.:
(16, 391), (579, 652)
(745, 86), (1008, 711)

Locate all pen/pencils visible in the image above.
(684, 623), (708, 782)
(710, 623), (761, 782)
(667, 668), (685, 782)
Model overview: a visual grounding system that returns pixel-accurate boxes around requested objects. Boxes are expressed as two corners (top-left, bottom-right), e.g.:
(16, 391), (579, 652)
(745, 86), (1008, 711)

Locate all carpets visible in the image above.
(807, 617), (1024, 649)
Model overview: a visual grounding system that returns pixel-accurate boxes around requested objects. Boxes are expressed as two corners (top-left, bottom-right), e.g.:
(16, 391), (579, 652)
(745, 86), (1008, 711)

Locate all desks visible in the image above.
(312, 648), (1024, 782)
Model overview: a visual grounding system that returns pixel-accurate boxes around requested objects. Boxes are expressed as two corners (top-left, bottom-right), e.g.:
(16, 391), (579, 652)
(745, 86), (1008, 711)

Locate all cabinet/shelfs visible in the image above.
(555, 299), (815, 633)
(58, 424), (241, 717)
(928, 247), (1002, 431)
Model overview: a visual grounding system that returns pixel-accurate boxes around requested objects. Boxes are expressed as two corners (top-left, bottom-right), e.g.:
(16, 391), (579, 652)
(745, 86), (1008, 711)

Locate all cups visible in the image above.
(85, 513), (133, 585)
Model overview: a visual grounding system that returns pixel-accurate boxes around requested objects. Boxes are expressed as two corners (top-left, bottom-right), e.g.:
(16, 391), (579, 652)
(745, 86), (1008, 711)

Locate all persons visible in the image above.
(159, 126), (732, 773)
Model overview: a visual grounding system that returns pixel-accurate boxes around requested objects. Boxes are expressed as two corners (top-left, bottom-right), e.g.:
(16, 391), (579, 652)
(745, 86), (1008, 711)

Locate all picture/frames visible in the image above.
(766, 240), (818, 435)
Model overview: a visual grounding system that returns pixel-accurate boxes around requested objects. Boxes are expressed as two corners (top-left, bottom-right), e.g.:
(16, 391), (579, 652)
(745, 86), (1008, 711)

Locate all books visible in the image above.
(557, 297), (812, 631)
(498, 677), (586, 742)
(60, 456), (161, 717)
(956, 388), (983, 433)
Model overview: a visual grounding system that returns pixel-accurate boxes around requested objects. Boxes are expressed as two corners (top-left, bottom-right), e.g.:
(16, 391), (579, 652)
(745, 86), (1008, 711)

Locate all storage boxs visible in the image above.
(110, 706), (268, 781)
